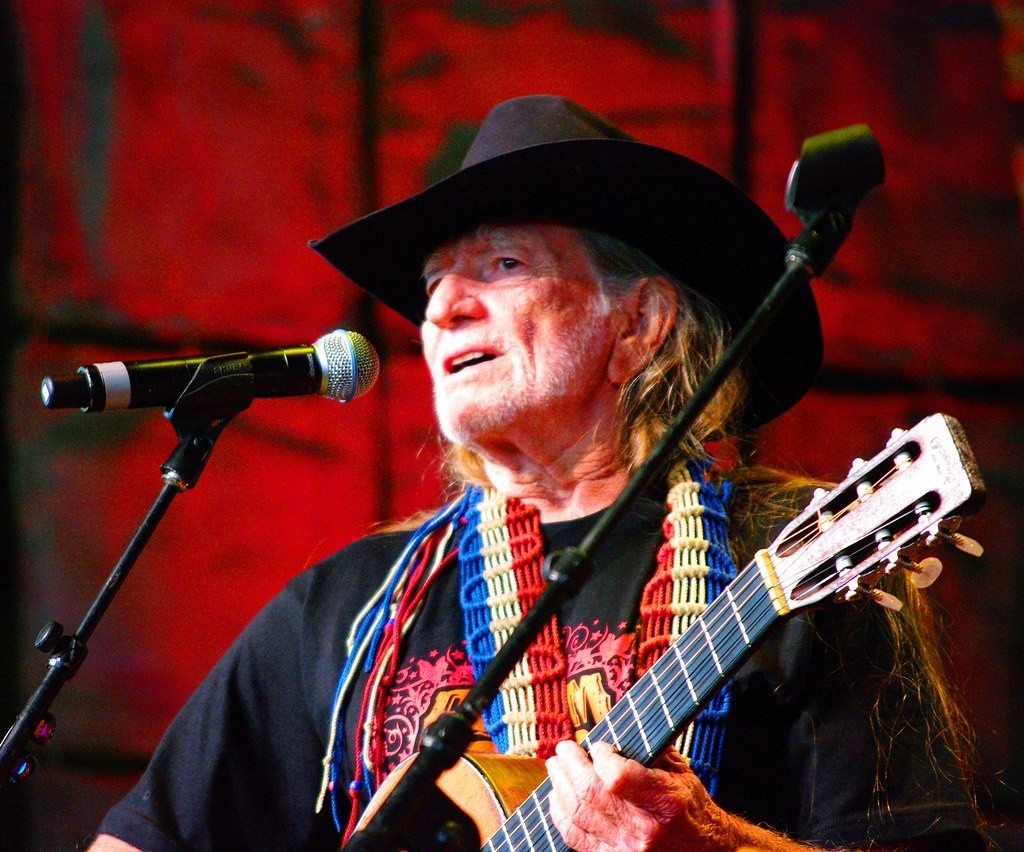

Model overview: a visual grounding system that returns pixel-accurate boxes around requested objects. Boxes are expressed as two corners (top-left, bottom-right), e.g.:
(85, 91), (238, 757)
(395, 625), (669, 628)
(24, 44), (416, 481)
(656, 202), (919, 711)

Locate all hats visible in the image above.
(308, 94), (824, 442)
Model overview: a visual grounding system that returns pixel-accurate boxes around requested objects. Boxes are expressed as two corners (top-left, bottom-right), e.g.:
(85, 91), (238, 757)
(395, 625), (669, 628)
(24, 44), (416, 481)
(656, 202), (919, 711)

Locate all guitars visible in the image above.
(473, 411), (987, 852)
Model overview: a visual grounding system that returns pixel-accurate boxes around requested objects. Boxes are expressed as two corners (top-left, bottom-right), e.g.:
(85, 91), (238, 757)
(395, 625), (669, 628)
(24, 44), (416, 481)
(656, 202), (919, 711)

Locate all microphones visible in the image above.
(41, 330), (379, 413)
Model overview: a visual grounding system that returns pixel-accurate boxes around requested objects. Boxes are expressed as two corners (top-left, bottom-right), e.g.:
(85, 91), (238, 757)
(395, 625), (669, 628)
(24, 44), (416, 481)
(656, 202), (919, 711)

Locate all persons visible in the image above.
(88, 94), (992, 852)
